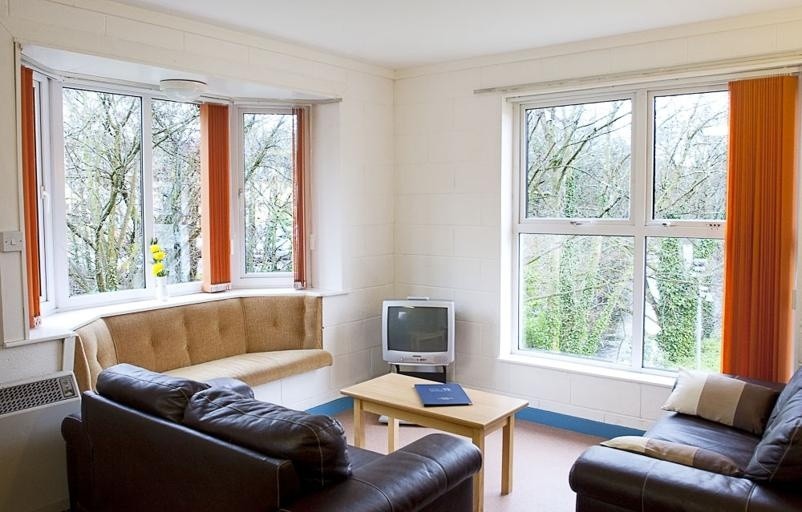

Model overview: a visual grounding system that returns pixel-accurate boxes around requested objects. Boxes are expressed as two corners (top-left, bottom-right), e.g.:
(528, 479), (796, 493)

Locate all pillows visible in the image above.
(601, 433), (744, 478)
(658, 365), (778, 437)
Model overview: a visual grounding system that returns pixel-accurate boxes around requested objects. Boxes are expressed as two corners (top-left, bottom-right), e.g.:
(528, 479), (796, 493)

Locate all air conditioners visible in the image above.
(1, 370), (84, 511)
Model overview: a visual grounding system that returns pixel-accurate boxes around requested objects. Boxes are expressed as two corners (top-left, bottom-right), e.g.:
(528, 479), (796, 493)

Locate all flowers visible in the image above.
(148, 237), (168, 276)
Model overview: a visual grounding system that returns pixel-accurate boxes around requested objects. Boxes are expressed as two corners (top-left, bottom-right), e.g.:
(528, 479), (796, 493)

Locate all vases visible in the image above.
(156, 275), (168, 301)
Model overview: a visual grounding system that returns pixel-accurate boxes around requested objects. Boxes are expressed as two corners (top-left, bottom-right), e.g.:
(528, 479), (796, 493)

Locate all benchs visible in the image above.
(71, 292), (332, 399)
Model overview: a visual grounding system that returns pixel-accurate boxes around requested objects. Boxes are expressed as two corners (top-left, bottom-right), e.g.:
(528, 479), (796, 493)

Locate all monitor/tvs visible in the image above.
(381, 300), (455, 364)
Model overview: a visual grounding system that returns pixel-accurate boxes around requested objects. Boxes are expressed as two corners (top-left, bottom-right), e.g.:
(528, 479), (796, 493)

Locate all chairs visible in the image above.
(567, 356), (802, 511)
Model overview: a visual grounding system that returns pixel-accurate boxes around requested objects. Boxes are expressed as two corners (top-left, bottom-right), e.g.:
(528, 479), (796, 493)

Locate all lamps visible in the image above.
(158, 79), (209, 104)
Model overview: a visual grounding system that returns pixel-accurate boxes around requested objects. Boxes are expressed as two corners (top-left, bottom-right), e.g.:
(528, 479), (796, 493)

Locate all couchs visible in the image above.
(59, 361), (483, 512)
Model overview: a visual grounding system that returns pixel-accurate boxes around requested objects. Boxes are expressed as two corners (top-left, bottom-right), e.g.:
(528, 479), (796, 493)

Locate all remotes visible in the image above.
(406, 296), (429, 300)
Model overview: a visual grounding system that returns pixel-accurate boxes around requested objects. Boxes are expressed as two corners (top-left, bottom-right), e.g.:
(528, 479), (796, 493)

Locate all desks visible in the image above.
(374, 358), (454, 428)
(340, 371), (532, 511)
